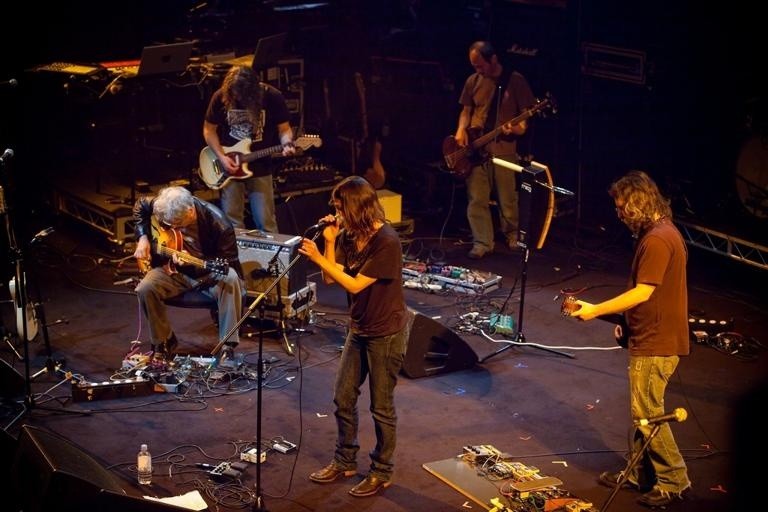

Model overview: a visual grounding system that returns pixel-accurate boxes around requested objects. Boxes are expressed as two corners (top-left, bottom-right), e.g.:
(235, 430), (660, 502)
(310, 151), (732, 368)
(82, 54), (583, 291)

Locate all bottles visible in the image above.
(137, 444), (152, 485)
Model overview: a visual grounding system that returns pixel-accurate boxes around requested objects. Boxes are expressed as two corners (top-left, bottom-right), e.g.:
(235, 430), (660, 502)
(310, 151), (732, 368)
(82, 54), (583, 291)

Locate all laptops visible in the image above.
(127, 41), (194, 80)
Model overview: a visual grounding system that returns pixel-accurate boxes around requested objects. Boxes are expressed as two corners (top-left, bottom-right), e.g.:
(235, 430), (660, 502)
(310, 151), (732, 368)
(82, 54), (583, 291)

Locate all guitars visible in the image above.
(0, 187), (39, 340)
(355, 71), (385, 188)
(135, 215), (228, 278)
(200, 135), (322, 189)
(440, 93), (557, 180)
(560, 295), (629, 347)
(322, 71), (343, 164)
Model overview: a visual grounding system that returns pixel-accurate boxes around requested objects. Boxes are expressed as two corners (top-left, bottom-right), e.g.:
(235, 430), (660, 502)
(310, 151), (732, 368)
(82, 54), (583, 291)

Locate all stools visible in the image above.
(164, 287), (246, 335)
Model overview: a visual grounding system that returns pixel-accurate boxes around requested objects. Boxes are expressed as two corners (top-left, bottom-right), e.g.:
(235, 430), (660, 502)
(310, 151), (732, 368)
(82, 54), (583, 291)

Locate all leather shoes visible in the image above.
(639, 487), (690, 506)
(309, 460), (357, 483)
(217, 350), (235, 370)
(600, 471), (638, 489)
(146, 332), (178, 371)
(349, 470), (393, 496)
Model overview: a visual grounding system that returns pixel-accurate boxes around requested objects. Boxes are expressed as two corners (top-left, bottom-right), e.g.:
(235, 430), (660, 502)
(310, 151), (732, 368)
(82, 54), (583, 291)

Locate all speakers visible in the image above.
(400, 300), (480, 381)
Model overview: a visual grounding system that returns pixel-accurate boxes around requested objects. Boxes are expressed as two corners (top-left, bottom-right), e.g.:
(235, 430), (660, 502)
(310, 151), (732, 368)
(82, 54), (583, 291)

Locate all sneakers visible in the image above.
(506, 239), (520, 252)
(468, 246), (494, 259)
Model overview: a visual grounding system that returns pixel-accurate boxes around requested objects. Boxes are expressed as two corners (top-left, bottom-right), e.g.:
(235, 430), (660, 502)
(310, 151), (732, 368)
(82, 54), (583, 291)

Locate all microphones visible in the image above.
(1, 149), (13, 168)
(315, 212), (343, 233)
(636, 409), (689, 432)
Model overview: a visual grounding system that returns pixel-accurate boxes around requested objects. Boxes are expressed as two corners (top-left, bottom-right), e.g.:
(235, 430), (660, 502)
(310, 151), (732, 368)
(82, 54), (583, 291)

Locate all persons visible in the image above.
(132, 181), (247, 373)
(201, 62), (297, 234)
(569, 172), (696, 508)
(297, 174), (413, 499)
(454, 37), (537, 259)
